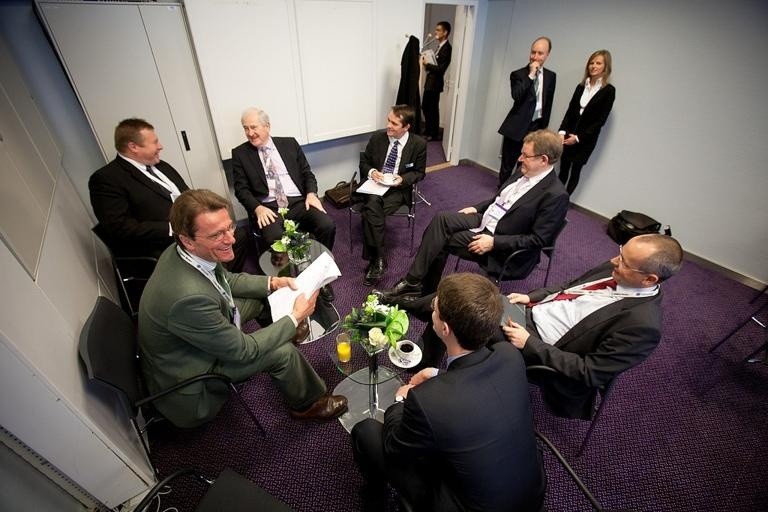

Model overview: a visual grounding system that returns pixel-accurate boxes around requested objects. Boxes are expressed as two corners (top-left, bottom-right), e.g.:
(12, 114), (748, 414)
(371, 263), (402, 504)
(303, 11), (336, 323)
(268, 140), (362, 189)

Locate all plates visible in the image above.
(388, 340), (423, 368)
(379, 171), (404, 187)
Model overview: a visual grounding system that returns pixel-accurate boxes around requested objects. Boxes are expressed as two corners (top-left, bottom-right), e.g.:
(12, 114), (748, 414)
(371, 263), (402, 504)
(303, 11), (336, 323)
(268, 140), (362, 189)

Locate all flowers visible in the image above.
(342, 295), (410, 355)
(278, 207), (313, 263)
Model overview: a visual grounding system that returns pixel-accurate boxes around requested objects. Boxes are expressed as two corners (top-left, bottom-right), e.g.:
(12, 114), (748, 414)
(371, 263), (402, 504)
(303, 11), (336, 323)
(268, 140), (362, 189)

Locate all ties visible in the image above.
(383, 140), (400, 175)
(469, 176), (529, 233)
(260, 145), (289, 210)
(527, 278), (616, 309)
(146, 165), (164, 184)
(532, 70), (540, 123)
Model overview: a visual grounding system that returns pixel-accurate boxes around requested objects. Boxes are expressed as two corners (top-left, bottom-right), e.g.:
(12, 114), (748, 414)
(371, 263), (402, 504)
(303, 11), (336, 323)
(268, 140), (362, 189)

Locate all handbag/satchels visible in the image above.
(325, 180), (359, 210)
(606, 210), (662, 246)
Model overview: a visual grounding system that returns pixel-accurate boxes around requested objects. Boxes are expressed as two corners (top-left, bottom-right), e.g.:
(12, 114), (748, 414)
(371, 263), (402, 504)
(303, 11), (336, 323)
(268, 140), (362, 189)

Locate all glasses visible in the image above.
(522, 152), (544, 159)
(618, 244), (655, 275)
(191, 223), (238, 242)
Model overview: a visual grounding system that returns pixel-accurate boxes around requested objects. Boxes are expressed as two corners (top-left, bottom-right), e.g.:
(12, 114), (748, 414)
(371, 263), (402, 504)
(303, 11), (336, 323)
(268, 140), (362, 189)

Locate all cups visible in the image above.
(381, 173), (394, 183)
(336, 333), (352, 363)
(397, 342), (415, 362)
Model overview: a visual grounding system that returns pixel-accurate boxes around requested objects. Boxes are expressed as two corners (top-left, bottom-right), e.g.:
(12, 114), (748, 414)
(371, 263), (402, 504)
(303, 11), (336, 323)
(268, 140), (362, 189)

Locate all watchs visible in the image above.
(393, 396), (405, 403)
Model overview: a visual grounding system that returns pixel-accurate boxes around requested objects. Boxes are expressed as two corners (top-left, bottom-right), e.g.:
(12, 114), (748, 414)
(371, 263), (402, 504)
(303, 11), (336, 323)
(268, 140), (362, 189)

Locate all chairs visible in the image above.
(78, 296), (266, 478)
(91, 222), (158, 321)
(323, 313), (424, 435)
(453, 216), (569, 289)
(132, 468), (292, 512)
(522, 365), (616, 459)
(348, 171), (417, 257)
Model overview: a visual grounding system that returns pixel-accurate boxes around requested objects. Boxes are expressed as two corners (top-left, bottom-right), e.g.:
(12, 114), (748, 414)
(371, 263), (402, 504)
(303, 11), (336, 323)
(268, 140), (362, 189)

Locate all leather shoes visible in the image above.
(288, 394), (348, 422)
(369, 279), (424, 306)
(363, 257), (388, 286)
(292, 321), (310, 344)
(320, 283), (335, 302)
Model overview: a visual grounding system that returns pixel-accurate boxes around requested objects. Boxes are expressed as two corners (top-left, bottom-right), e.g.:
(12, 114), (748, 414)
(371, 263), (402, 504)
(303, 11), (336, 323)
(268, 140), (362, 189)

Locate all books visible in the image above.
(422, 49), (438, 66)
(267, 251), (341, 323)
(355, 178), (391, 197)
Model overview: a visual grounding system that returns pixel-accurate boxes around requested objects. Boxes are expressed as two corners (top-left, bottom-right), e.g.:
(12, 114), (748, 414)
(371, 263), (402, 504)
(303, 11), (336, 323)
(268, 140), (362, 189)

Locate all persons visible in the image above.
(559, 50), (615, 196)
(421, 21), (451, 142)
(371, 129), (570, 301)
(370, 232), (683, 422)
(232, 108), (336, 303)
(497, 37), (556, 194)
(136, 188), (347, 426)
(350, 271), (548, 512)
(360, 104), (428, 287)
(87, 117), (194, 268)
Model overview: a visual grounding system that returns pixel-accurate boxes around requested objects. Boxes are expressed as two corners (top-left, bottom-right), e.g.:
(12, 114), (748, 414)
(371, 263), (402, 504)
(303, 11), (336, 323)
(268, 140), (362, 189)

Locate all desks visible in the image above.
(259, 239), (340, 346)
